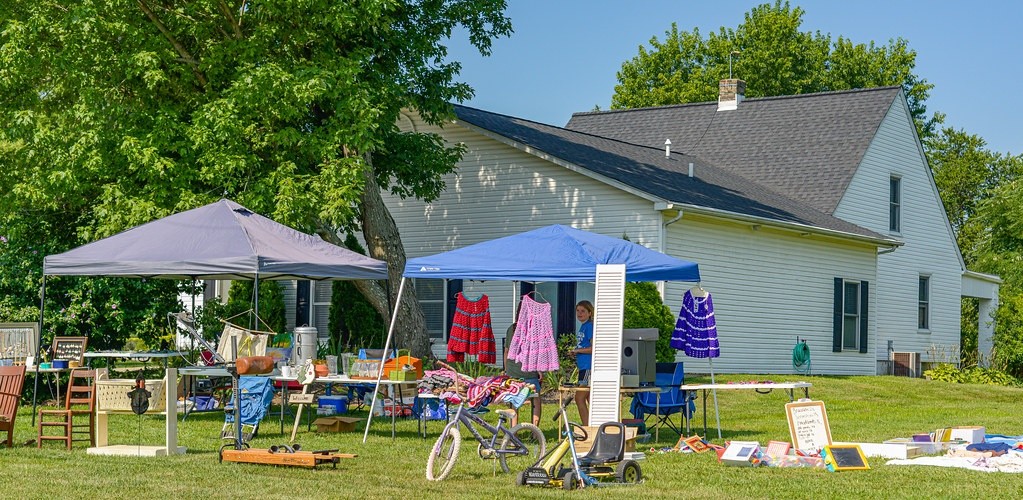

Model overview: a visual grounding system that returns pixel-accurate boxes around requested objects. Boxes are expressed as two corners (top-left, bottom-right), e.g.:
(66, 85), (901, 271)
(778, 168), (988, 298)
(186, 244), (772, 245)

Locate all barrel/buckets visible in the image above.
(292, 323), (319, 365)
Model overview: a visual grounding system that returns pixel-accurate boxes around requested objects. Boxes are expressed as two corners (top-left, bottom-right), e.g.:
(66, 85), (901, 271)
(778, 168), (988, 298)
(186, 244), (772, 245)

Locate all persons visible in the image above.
(564, 300), (593, 426)
(504, 322), (543, 449)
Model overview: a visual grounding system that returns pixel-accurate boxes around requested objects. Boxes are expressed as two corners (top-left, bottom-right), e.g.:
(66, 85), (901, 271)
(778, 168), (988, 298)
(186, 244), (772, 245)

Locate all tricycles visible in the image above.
(516, 420), (642, 490)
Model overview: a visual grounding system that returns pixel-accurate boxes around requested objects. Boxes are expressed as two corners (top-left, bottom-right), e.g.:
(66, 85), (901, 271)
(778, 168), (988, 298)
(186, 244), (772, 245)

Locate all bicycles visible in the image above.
(424, 360), (548, 483)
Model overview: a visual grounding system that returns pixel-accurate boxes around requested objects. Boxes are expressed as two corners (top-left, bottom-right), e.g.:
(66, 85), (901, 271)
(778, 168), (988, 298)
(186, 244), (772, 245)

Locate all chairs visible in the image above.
(629, 362), (698, 436)
(38, 369), (96, 449)
(0, 365), (26, 447)
(342, 349), (394, 413)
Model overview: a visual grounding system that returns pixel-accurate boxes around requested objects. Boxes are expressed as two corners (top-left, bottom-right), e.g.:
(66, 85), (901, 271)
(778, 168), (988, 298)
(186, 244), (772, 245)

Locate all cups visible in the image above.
(277, 361), (297, 377)
(341, 353), (354, 374)
(325, 355), (338, 376)
(326, 383), (332, 396)
(358, 362), (378, 377)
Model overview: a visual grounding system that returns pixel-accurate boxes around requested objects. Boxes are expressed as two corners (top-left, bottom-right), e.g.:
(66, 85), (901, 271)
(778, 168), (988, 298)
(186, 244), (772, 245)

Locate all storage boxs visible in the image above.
(52, 360), (70, 369)
(317, 394), (349, 413)
(720, 441), (762, 467)
(311, 416), (366, 433)
(884, 440), (945, 454)
(950, 426), (986, 443)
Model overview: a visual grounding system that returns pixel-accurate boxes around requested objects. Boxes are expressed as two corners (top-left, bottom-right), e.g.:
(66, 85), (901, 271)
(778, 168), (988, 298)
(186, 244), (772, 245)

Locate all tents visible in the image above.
(31, 196), (406, 427)
(362, 223), (721, 447)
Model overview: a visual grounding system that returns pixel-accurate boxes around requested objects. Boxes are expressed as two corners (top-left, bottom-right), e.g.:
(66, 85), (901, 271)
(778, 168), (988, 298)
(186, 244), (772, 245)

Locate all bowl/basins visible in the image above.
(42, 363), (51, 368)
(52, 359), (69, 369)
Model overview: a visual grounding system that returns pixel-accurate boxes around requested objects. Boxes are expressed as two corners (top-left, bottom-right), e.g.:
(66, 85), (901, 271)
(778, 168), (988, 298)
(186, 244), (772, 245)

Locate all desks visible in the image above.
(26, 366), (91, 409)
(417, 393), (539, 440)
(178, 368), (232, 421)
(558, 386), (661, 444)
(83, 351), (189, 399)
(679, 381), (811, 441)
(272, 375), (424, 441)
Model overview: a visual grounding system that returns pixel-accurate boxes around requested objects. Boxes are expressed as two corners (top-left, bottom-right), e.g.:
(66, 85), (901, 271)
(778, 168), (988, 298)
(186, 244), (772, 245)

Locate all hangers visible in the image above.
(516, 285), (547, 302)
(690, 281), (707, 295)
(454, 281), (482, 298)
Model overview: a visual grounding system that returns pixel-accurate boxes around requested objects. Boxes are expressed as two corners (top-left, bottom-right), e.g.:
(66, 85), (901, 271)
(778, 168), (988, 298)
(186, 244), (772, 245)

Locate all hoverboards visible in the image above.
(553, 395), (647, 490)
